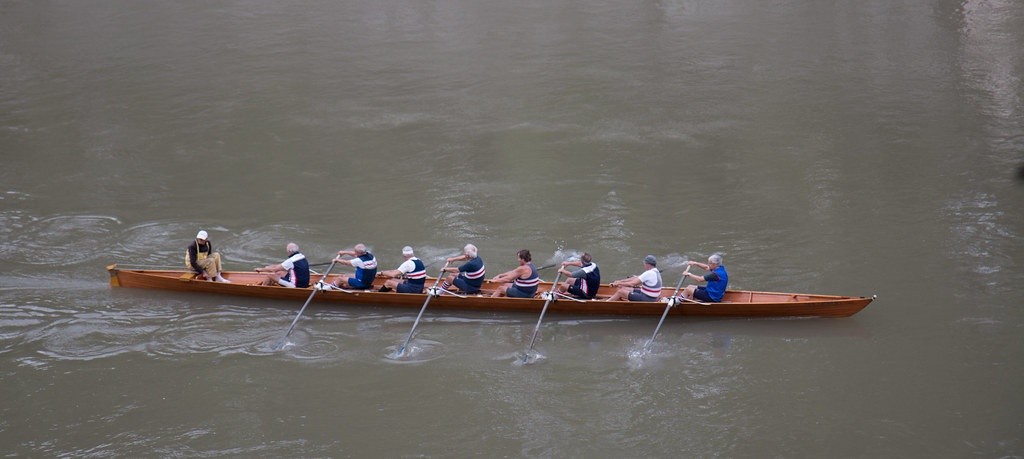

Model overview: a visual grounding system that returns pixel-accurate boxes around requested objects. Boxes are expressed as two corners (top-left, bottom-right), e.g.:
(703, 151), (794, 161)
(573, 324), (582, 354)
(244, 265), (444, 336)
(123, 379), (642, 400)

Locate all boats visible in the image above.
(105, 263), (877, 319)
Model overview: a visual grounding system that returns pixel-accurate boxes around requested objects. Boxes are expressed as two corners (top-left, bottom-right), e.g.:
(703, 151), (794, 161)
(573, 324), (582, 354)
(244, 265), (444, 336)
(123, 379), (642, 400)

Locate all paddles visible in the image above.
(255, 260), (338, 273)
(488, 263), (556, 284)
(523, 264), (566, 363)
(395, 260), (450, 357)
(610, 269), (664, 288)
(645, 263), (692, 350)
(282, 253), (342, 341)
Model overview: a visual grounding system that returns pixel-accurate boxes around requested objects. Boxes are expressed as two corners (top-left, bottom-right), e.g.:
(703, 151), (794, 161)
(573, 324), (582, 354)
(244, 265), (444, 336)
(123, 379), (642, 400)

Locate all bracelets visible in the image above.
(443, 268), (446, 272)
(381, 271), (384, 276)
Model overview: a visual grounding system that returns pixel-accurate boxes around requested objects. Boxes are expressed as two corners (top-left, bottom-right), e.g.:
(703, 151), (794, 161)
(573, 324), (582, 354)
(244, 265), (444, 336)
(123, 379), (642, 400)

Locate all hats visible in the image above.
(197, 230), (209, 240)
(644, 255), (657, 266)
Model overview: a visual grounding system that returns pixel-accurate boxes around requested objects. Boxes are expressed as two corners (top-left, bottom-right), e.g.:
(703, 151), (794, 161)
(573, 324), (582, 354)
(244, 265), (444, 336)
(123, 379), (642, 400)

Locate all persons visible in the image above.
(323, 244), (377, 289)
(375, 245), (427, 295)
(489, 249), (539, 298)
(429, 244), (485, 295)
(254, 243), (310, 289)
(553, 252), (601, 300)
(607, 255), (662, 303)
(185, 230), (230, 283)
(676, 255), (729, 303)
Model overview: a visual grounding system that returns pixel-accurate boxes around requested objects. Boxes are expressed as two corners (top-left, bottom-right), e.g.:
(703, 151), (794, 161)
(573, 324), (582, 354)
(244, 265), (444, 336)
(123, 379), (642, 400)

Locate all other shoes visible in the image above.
(216, 276), (231, 283)
(313, 282), (331, 290)
(542, 291), (558, 300)
(427, 288), (442, 295)
(364, 289), (377, 292)
